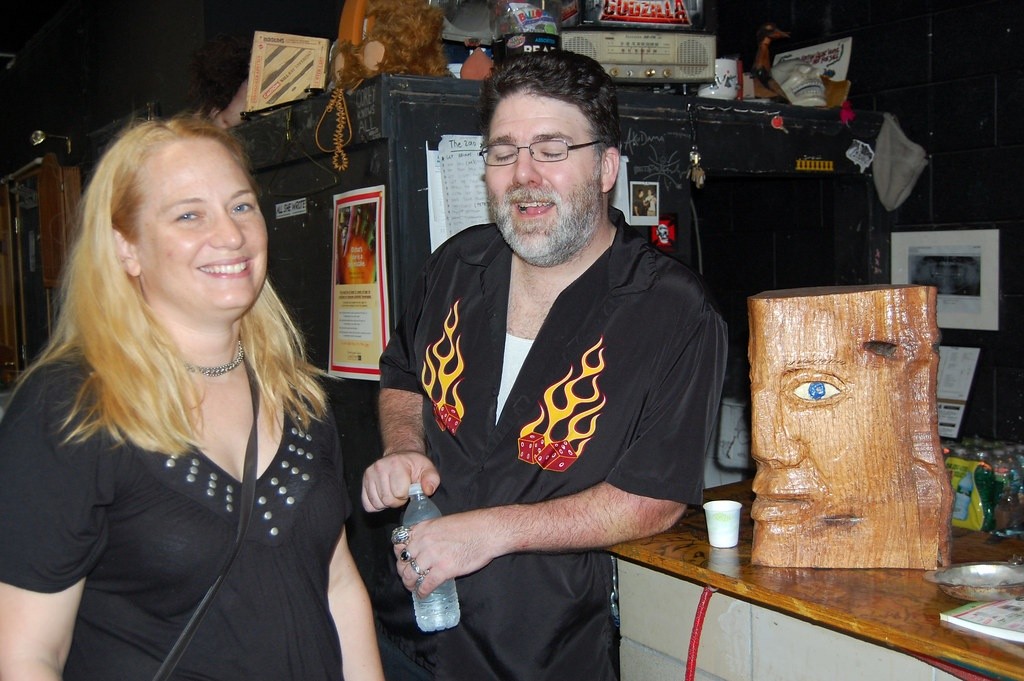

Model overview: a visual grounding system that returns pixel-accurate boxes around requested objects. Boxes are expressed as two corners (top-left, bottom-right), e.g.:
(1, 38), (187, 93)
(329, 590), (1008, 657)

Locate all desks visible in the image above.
(606, 485), (1023, 681)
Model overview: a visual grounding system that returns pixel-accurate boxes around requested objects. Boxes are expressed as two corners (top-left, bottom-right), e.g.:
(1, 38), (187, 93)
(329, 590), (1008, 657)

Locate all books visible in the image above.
(940, 600), (1024, 642)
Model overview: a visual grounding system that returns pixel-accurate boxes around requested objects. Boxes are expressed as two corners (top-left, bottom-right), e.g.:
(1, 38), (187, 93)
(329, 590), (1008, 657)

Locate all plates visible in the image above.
(923, 561), (1024, 602)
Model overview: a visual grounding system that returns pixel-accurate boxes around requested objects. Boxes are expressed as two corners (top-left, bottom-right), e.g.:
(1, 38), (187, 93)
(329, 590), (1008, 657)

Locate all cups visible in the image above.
(703, 500), (743, 549)
(697, 59), (738, 100)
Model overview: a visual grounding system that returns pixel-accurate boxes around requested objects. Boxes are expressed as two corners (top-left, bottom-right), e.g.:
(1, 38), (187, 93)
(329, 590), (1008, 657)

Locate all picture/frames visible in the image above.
(888, 221), (1003, 335)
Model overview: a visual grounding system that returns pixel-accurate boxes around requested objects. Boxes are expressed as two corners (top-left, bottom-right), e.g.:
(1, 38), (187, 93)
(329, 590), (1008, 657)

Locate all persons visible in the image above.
(0, 115), (382, 681)
(360, 51), (730, 681)
(750, 286), (948, 567)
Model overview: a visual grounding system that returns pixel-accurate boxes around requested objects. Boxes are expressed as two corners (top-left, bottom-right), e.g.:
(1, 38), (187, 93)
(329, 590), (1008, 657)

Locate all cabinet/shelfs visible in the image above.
(232, 80), (891, 484)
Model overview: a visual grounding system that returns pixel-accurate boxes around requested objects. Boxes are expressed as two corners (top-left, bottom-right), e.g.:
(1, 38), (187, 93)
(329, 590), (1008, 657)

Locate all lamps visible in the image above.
(30, 129), (72, 155)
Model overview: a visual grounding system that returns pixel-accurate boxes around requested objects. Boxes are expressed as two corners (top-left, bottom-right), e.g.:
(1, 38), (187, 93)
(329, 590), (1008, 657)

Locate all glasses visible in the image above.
(479, 140), (607, 166)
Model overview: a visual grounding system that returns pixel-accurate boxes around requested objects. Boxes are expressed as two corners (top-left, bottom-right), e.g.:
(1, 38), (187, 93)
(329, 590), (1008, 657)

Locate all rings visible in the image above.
(399, 549), (415, 563)
(391, 526), (411, 544)
(410, 560), (424, 573)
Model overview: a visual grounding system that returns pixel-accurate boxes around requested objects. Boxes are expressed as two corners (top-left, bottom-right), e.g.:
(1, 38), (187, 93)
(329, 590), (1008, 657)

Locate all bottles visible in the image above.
(403, 482), (460, 632)
(939, 437), (1024, 536)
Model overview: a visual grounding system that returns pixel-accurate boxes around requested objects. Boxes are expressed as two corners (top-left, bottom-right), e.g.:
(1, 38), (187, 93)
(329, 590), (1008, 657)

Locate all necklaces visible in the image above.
(180, 337), (246, 376)
